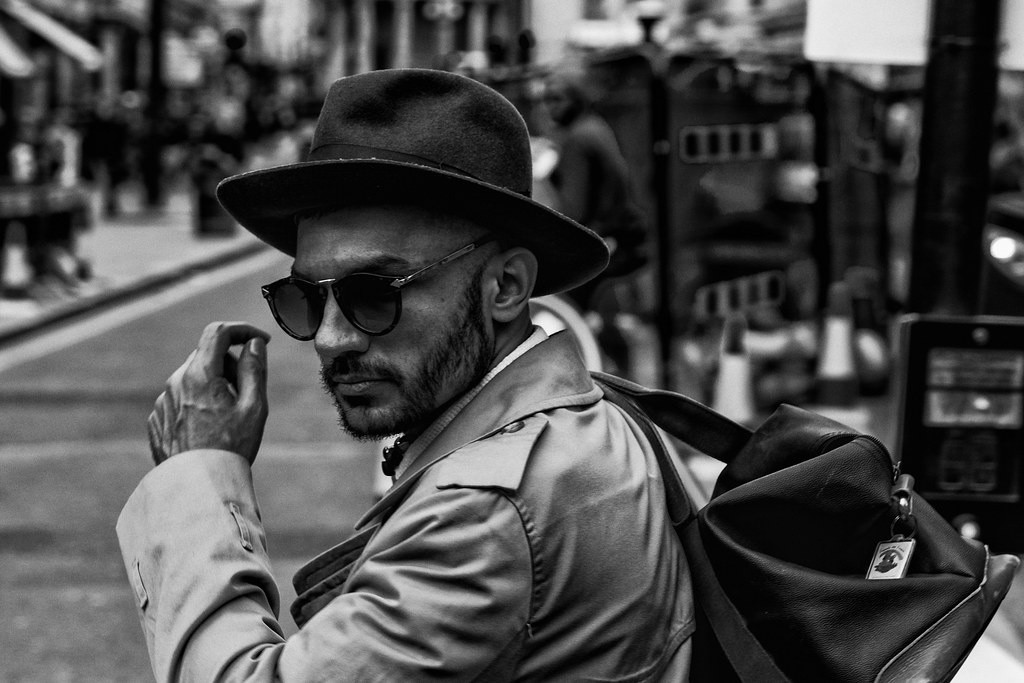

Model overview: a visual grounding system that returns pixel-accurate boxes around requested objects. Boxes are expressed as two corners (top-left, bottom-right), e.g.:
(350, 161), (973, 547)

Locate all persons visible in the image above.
(112, 67), (699, 683)
(538, 1), (906, 494)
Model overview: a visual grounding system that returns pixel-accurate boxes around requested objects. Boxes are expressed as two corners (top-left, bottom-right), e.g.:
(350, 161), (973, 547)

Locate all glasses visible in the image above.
(262, 236), (499, 336)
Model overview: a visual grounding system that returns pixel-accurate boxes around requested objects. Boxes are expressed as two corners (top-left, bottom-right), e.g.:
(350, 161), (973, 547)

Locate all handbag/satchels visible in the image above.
(587, 369), (1020, 683)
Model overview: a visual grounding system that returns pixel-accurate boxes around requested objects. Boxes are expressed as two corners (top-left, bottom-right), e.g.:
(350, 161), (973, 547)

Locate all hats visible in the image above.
(215, 68), (609, 297)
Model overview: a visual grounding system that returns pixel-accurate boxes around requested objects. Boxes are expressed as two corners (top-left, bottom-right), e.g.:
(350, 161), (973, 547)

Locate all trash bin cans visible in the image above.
(894, 319), (1024, 559)
(192, 169), (236, 238)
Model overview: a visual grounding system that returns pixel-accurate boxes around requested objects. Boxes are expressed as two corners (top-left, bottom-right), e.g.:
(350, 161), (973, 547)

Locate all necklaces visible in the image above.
(381, 432), (417, 477)
